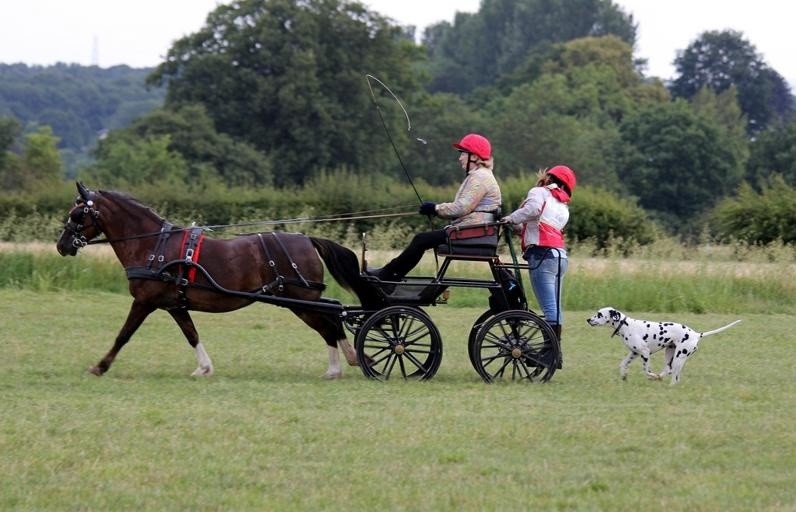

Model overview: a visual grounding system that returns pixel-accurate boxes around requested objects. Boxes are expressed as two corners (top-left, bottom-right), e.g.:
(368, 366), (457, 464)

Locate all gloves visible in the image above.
(419, 202), (436, 216)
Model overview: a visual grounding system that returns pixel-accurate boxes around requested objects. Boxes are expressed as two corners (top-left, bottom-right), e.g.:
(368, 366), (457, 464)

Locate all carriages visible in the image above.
(57, 181), (559, 383)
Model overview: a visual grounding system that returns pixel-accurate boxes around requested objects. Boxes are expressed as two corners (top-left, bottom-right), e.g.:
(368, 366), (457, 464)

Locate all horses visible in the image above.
(54, 179), (360, 381)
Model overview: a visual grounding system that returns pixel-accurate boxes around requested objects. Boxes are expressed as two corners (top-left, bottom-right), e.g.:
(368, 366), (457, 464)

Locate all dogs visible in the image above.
(583, 304), (743, 388)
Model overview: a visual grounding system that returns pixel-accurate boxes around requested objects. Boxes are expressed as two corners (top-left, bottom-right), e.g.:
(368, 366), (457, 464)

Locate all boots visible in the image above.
(363, 247), (420, 295)
(524, 324), (562, 368)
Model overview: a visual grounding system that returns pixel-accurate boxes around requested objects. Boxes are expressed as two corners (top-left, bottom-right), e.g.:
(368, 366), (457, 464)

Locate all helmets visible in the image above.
(453, 134), (491, 160)
(547, 165), (576, 197)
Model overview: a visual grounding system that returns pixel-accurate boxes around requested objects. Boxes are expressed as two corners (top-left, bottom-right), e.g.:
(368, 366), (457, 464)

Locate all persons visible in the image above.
(500, 166), (577, 370)
(365, 133), (501, 294)
